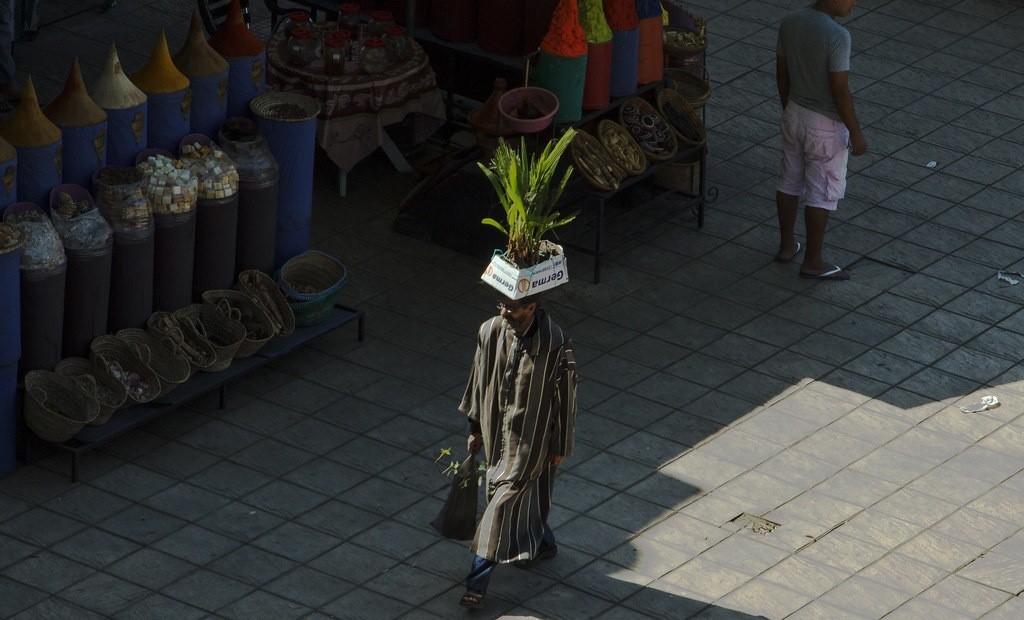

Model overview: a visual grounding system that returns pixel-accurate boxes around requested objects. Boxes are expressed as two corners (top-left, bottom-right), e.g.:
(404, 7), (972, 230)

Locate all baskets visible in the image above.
(563, 64), (710, 191)
(250, 91), (321, 121)
(23, 248), (353, 443)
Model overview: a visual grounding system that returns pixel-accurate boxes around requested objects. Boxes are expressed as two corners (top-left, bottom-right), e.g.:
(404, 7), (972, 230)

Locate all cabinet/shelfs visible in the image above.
(520, 44), (708, 286)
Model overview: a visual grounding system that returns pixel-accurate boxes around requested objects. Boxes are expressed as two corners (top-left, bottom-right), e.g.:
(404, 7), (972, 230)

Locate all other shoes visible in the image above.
(514, 544), (557, 569)
(459, 590), (485, 610)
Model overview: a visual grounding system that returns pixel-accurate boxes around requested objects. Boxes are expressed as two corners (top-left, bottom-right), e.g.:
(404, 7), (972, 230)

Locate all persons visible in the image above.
(458, 290), (576, 609)
(775, 0), (867, 280)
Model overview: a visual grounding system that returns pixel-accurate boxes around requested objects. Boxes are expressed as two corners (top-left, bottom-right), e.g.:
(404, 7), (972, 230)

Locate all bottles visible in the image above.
(283, 3), (407, 76)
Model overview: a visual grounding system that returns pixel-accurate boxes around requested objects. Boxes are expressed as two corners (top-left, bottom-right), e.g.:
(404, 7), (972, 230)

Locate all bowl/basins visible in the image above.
(497, 87), (560, 133)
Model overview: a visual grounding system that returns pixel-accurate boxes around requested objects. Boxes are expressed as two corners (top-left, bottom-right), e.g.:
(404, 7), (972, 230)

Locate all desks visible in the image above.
(265, 10), (448, 199)
(16, 302), (365, 483)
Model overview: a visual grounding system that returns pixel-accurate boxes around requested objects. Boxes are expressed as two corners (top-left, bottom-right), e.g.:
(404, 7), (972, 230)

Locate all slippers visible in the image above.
(776, 234), (802, 262)
(799, 263), (849, 281)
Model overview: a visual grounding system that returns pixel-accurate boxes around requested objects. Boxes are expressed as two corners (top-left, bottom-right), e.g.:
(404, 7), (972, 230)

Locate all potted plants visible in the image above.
(476, 127), (582, 302)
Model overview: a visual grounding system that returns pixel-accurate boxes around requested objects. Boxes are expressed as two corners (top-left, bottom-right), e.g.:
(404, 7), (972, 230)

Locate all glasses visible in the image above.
(496, 299), (528, 312)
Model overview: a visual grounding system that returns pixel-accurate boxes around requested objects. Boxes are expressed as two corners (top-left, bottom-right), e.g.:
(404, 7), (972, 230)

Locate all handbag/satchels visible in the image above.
(430, 453), (477, 540)
